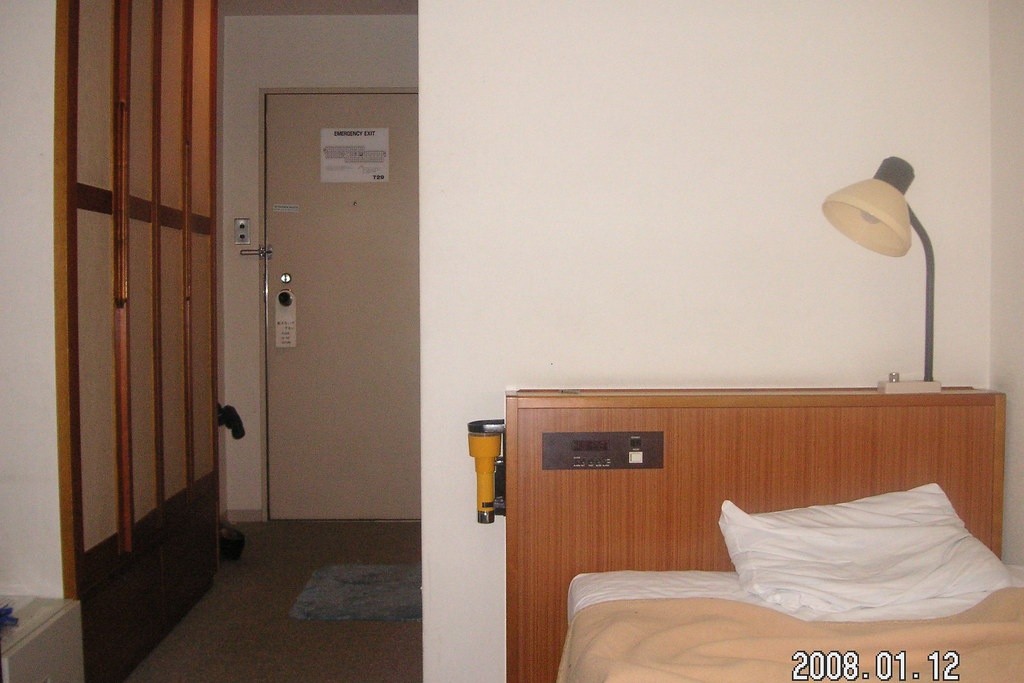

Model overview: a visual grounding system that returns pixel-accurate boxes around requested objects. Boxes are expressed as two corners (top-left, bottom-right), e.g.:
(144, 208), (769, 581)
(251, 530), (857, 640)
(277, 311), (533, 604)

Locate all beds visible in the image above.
(553, 570), (1024, 682)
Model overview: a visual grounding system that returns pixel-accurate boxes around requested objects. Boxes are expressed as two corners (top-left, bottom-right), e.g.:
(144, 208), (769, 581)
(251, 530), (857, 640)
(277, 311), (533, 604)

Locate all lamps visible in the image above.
(821, 157), (941, 394)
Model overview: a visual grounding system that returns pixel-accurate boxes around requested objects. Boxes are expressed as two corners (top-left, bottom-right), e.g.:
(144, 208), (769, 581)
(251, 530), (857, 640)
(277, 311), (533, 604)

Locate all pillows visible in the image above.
(718, 483), (1014, 615)
